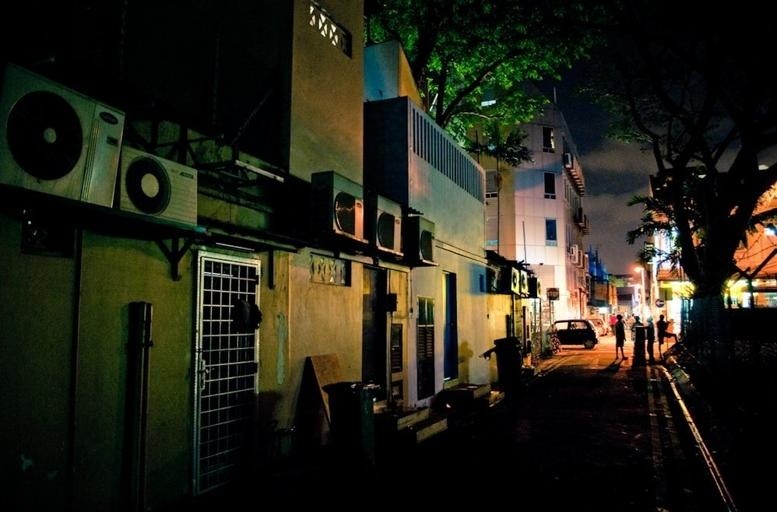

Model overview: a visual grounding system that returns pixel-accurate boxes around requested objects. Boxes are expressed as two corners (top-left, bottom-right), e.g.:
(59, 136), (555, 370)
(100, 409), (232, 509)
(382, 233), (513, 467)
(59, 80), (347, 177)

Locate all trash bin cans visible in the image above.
(494, 336), (524, 384)
(322, 380), (382, 458)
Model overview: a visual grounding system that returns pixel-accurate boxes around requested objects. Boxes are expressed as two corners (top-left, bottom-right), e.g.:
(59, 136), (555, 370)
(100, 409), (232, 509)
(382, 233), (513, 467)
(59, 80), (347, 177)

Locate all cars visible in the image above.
(587, 317), (609, 337)
(543, 318), (599, 350)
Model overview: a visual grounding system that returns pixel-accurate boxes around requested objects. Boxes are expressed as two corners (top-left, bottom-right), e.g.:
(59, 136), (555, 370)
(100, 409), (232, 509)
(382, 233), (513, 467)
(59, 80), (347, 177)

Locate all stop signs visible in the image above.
(546, 288), (559, 300)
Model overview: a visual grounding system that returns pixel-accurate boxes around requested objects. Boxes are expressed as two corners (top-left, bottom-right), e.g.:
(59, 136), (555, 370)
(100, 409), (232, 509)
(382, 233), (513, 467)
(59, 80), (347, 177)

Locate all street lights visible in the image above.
(633, 265), (645, 310)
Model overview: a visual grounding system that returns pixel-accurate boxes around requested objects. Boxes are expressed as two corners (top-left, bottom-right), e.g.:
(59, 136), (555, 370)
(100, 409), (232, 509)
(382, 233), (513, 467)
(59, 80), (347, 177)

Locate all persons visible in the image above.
(614, 314), (628, 360)
(655, 314), (678, 359)
(642, 316), (655, 362)
(608, 312), (644, 341)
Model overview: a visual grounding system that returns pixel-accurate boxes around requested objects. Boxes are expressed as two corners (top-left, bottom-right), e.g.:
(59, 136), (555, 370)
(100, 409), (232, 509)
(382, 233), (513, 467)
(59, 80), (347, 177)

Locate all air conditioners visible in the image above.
(506, 262), (542, 300)
(310, 170), (435, 270)
(0, 63), (198, 225)
